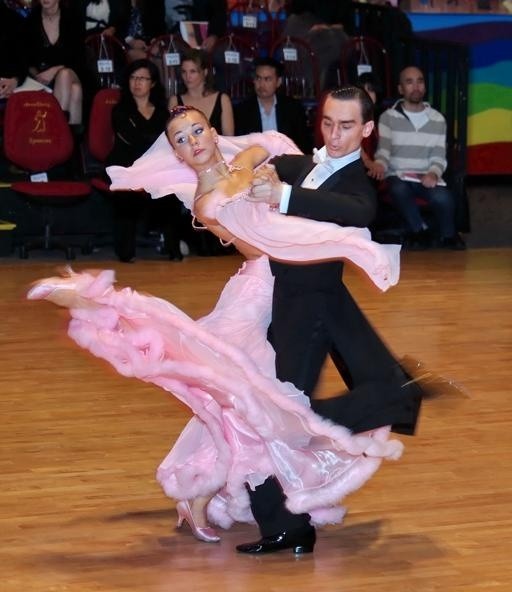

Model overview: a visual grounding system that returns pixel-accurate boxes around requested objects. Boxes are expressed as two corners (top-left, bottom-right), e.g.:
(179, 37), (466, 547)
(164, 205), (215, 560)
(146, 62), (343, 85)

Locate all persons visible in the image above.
(236, 84), (422, 555)
(1, 0), (448, 263)
(26, 105), (403, 543)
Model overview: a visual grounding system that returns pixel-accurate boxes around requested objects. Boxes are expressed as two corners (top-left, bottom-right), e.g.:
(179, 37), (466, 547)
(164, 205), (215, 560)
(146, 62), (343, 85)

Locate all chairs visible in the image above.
(1, 1), (390, 262)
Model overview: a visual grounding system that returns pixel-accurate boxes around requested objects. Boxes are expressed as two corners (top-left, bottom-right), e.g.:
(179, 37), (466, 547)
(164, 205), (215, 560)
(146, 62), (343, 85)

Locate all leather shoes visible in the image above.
(235, 525), (316, 554)
(410, 231), (466, 250)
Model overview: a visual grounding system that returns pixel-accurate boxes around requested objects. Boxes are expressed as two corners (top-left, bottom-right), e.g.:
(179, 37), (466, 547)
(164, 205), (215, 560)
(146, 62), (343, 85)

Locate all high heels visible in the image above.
(25, 265), (95, 301)
(176, 499), (221, 543)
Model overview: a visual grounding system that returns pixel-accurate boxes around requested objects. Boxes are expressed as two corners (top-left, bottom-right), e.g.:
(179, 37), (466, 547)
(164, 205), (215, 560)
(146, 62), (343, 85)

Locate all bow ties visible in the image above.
(312, 146), (334, 173)
